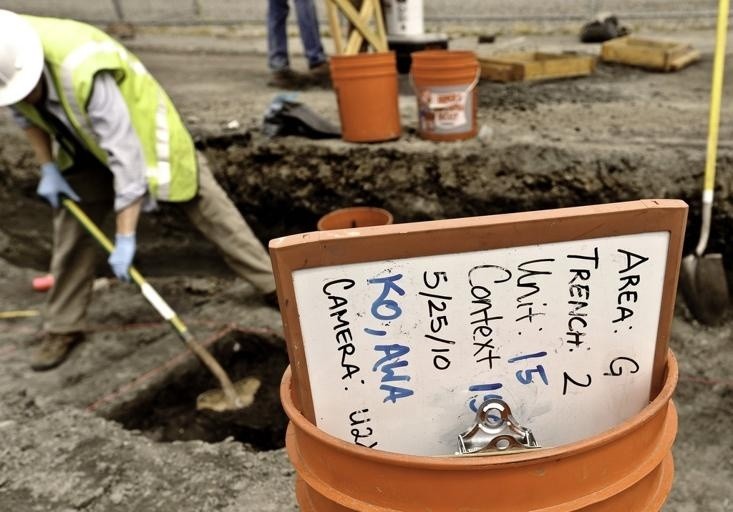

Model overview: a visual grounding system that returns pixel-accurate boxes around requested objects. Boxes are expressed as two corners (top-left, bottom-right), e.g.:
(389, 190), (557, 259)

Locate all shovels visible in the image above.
(678, 0), (730, 329)
(57, 191), (261, 412)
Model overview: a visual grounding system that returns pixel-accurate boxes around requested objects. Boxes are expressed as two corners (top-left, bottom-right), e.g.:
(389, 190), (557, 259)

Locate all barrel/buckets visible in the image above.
(317, 206), (394, 231)
(406, 50), (480, 141)
(328, 53), (400, 141)
(281, 349), (679, 512)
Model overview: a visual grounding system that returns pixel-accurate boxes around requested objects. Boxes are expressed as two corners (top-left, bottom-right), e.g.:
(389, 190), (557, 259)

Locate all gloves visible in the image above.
(106, 231), (138, 287)
(35, 161), (82, 209)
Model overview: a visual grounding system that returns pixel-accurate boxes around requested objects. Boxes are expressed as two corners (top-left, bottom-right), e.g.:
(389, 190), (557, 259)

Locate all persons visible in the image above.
(263, 1), (331, 80)
(0, 7), (283, 371)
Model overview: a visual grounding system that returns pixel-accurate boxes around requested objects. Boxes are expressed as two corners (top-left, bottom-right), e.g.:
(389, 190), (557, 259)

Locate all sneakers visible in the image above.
(269, 61), (330, 89)
(29, 330), (86, 370)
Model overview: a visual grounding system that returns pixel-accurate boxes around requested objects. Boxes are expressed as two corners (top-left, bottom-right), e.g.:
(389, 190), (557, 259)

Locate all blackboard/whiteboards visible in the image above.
(267, 198), (689, 456)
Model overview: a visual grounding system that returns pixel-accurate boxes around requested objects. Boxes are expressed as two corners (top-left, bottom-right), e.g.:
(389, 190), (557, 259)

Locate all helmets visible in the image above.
(0, 9), (46, 109)
